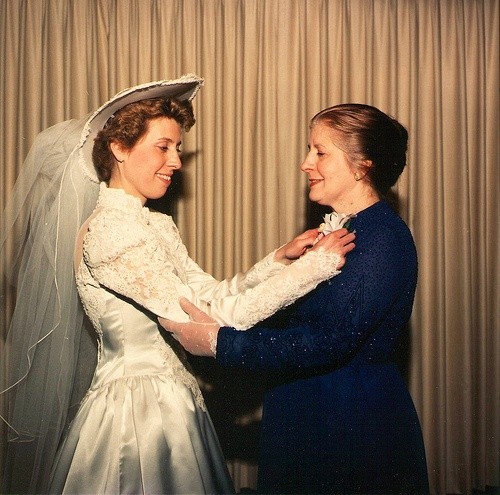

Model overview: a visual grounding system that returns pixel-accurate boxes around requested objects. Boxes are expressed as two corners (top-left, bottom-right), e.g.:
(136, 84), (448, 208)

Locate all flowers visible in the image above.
(317, 211), (357, 236)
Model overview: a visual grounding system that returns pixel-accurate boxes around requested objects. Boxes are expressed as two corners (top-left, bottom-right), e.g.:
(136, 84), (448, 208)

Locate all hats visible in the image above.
(79, 73), (204, 183)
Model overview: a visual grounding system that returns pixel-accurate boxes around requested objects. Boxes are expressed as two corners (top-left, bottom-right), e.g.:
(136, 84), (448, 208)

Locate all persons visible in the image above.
(0, 73), (355, 495)
(157, 104), (429, 495)
